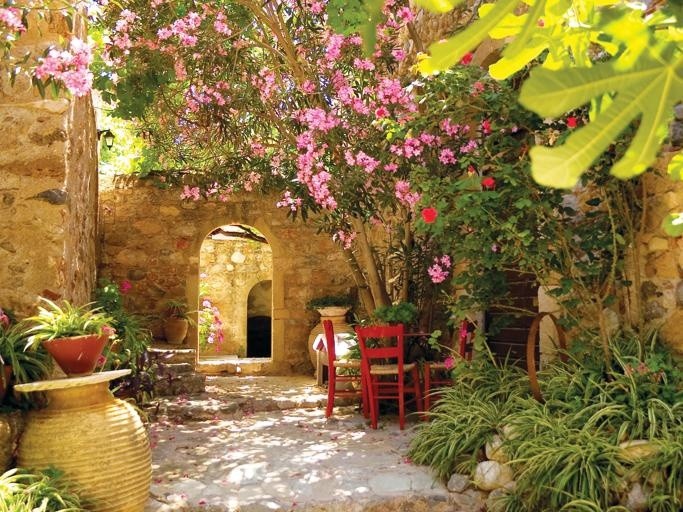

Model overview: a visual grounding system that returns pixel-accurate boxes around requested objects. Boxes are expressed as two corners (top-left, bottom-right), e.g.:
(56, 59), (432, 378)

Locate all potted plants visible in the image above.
(373, 301), (418, 333)
(14, 295), (115, 378)
(160, 300), (193, 346)
(305, 294), (357, 318)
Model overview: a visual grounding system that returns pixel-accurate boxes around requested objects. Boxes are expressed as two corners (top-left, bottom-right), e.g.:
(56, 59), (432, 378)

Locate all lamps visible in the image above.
(96, 128), (116, 151)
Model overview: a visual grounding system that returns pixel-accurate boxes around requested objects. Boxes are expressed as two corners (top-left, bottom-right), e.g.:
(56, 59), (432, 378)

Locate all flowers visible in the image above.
(198, 298), (225, 354)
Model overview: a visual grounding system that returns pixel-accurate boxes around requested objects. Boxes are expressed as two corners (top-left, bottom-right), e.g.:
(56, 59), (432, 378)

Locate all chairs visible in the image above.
(321, 316), (478, 435)
